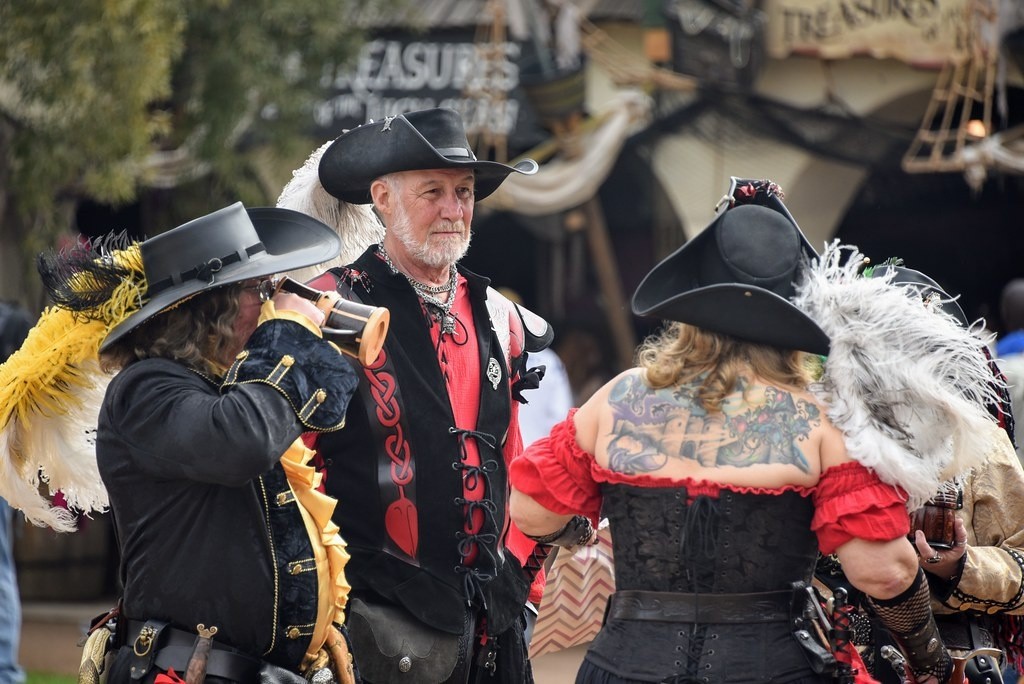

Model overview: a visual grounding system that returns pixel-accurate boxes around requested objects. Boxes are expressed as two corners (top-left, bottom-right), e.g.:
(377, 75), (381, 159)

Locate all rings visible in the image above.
(924, 551), (941, 564)
(954, 539), (967, 545)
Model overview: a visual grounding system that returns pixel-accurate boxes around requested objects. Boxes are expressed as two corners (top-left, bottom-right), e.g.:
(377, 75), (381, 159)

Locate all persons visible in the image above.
(300, 107), (546, 684)
(77, 199), (352, 684)
(0, 304), (29, 684)
(509, 175), (1024, 684)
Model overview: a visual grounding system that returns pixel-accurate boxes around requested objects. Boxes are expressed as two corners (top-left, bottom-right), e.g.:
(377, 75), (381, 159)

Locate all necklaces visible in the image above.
(377, 239), (459, 336)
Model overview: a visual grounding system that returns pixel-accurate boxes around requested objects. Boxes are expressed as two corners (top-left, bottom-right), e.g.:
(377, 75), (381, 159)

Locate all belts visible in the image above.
(609, 591), (798, 625)
(112, 612), (313, 684)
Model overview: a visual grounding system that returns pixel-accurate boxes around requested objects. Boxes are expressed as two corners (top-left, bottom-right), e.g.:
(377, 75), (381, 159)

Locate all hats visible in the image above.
(0, 200), (342, 536)
(631, 178), (1010, 510)
(278, 108), (539, 281)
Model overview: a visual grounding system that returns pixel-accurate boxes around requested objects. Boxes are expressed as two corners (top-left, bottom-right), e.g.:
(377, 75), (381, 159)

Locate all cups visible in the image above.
(907, 489), (957, 549)
(271, 276), (390, 367)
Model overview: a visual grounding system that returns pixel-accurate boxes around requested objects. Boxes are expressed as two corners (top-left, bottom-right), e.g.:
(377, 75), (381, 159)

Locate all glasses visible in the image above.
(232, 279), (281, 305)
